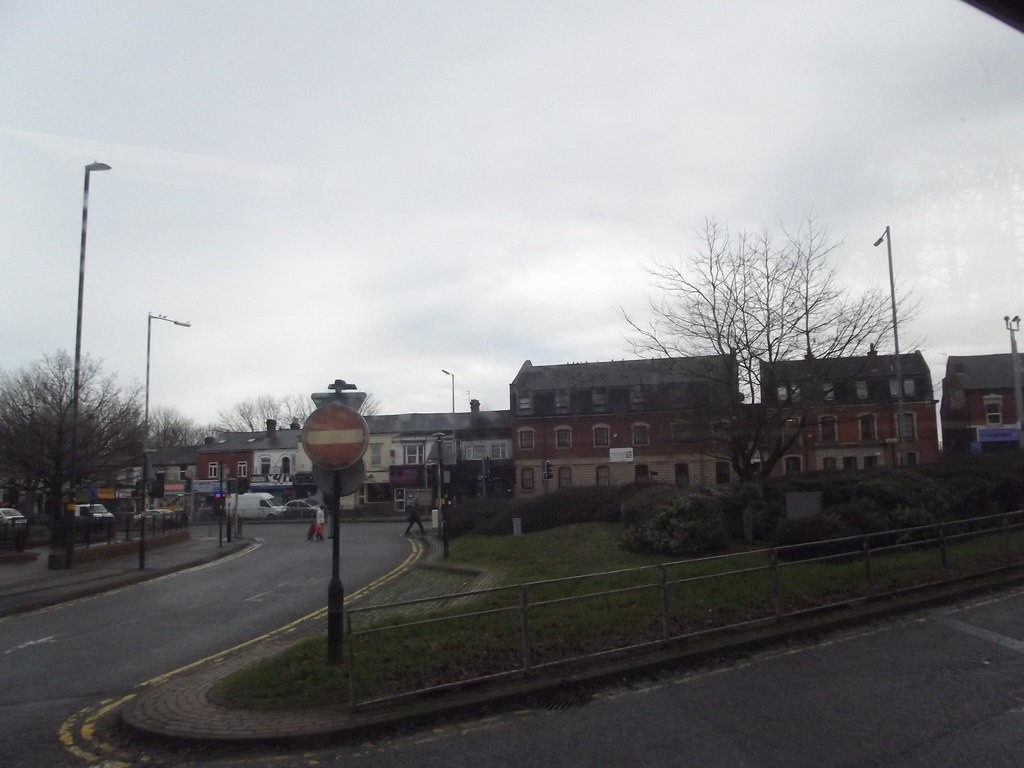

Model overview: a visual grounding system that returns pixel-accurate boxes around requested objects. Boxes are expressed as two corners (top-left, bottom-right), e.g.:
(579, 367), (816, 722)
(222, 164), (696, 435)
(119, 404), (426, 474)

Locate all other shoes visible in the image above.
(315, 535), (318, 539)
(320, 537), (325, 540)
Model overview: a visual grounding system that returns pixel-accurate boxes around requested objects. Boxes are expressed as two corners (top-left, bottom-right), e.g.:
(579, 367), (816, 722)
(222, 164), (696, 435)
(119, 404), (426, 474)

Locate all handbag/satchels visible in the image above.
(407, 516), (413, 522)
(316, 524), (322, 534)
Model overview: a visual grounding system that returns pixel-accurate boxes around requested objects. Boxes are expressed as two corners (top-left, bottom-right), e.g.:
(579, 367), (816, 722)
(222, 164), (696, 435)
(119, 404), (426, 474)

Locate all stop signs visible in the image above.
(301, 402), (370, 471)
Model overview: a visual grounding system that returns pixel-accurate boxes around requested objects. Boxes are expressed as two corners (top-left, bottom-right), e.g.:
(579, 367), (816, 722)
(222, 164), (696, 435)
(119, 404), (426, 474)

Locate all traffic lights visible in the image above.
(544, 460), (553, 480)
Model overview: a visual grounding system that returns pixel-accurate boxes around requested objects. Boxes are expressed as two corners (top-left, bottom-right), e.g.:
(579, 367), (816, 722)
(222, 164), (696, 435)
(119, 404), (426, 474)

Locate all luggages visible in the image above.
(307, 521), (317, 540)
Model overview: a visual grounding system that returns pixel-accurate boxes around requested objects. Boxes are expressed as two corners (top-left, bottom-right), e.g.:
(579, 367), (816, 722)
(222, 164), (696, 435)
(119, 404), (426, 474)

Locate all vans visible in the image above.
(225, 492), (288, 520)
(75, 502), (116, 520)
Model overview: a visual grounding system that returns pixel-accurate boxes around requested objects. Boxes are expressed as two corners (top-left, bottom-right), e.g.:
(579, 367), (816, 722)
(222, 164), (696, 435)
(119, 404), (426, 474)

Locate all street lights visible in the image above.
(139, 315), (192, 569)
(875, 226), (907, 466)
(442, 369), (456, 455)
(65, 163), (111, 567)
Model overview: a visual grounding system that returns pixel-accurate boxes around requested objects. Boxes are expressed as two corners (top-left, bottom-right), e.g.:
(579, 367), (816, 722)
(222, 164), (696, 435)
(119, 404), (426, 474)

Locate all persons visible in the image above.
(315, 505), (325, 539)
(405, 498), (425, 535)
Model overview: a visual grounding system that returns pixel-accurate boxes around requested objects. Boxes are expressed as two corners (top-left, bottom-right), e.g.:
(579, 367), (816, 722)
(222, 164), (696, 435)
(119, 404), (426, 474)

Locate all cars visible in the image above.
(133, 509), (176, 520)
(0, 508), (28, 527)
(283, 498), (328, 519)
